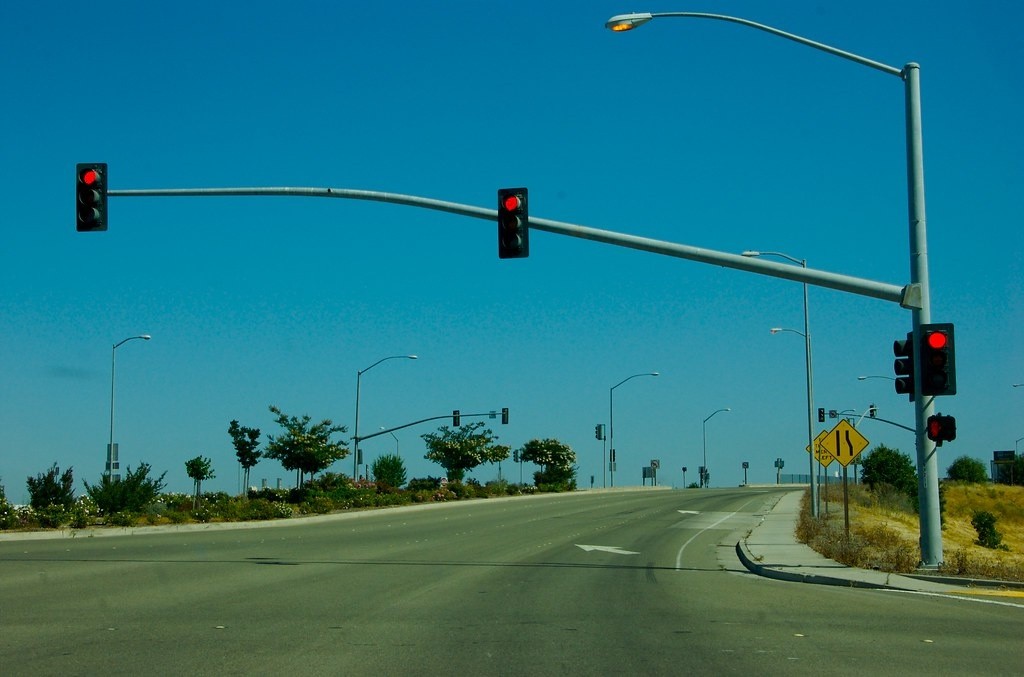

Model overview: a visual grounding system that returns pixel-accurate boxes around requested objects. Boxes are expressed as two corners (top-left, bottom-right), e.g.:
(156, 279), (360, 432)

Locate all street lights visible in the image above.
(379, 427), (399, 458)
(605, 11), (945, 567)
(108, 335), (150, 483)
(743, 250), (819, 516)
(704, 408), (731, 488)
(770, 327), (818, 516)
(353, 355), (417, 482)
(610, 373), (660, 488)
(837, 409), (856, 480)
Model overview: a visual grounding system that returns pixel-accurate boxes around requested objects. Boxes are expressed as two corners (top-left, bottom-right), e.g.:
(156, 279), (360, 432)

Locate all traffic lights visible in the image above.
(893, 329), (917, 402)
(595, 424), (602, 440)
(513, 450), (519, 462)
(919, 323), (957, 396)
(496, 186), (530, 258)
(610, 449), (616, 462)
(74, 163), (108, 231)
(928, 415), (957, 442)
(869, 405), (877, 417)
(818, 408), (826, 422)
